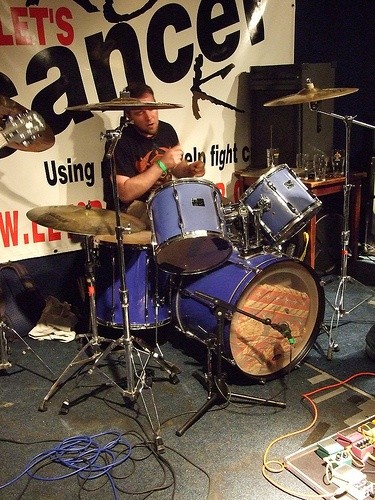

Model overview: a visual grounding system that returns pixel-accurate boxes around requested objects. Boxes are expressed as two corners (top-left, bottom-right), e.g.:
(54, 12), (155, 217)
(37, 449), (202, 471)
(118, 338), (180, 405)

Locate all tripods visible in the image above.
(37, 111), (287, 435)
(310, 104), (375, 360)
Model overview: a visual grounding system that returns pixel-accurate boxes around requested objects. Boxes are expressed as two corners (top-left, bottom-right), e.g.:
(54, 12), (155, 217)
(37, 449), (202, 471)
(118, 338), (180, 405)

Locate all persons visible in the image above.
(111, 82), (206, 225)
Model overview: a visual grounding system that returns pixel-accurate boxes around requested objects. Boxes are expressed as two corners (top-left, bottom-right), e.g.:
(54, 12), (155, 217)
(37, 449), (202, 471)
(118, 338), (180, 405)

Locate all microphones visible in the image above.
(279, 321), (294, 344)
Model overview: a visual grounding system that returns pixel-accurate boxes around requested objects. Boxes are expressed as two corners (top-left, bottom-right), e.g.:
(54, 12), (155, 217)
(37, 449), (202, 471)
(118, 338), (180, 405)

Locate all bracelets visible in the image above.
(158, 160), (167, 172)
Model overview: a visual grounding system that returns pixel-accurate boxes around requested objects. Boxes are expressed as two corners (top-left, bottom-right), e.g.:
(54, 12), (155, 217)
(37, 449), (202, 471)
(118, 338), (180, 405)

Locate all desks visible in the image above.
(240, 165), (362, 272)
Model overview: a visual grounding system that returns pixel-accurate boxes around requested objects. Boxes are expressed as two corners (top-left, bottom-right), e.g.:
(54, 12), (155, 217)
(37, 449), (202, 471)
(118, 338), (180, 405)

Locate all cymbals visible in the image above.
(26, 200), (147, 235)
(0, 96), (56, 154)
(263, 86), (359, 107)
(64, 97), (184, 110)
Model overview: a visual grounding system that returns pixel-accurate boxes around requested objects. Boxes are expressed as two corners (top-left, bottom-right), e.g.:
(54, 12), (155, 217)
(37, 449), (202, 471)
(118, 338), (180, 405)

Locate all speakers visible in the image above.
(248, 63), (335, 169)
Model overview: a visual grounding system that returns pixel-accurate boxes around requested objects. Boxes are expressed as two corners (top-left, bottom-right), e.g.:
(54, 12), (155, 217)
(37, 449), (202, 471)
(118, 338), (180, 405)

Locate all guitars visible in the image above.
(0, 110), (47, 149)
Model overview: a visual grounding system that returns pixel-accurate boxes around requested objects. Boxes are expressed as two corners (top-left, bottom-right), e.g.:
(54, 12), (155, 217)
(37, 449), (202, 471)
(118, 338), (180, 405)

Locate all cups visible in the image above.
(313, 154), (327, 181)
(266, 147), (279, 167)
(295, 152), (308, 180)
(332, 150), (345, 178)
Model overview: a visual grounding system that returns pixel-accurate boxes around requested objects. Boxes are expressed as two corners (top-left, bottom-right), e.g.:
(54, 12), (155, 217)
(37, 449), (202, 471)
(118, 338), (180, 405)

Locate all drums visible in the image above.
(239, 164), (322, 247)
(95, 230), (175, 330)
(146, 177), (233, 275)
(175, 248), (325, 381)
